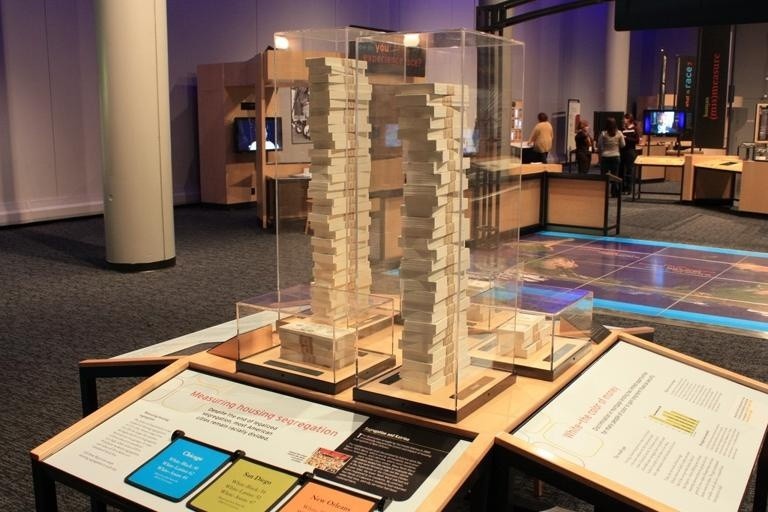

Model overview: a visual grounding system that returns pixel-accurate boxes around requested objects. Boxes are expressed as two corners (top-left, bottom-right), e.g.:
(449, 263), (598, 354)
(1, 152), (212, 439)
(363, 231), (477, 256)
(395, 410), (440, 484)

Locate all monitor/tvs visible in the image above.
(233, 117), (282, 152)
(593, 110), (624, 131)
(644, 110), (688, 137)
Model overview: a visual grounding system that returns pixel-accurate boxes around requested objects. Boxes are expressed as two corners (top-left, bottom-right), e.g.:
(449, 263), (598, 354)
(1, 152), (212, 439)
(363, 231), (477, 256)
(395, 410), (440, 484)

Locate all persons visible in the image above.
(651, 113), (676, 134)
(573, 120), (595, 176)
(617, 112), (642, 194)
(526, 111), (554, 165)
(594, 116), (627, 199)
